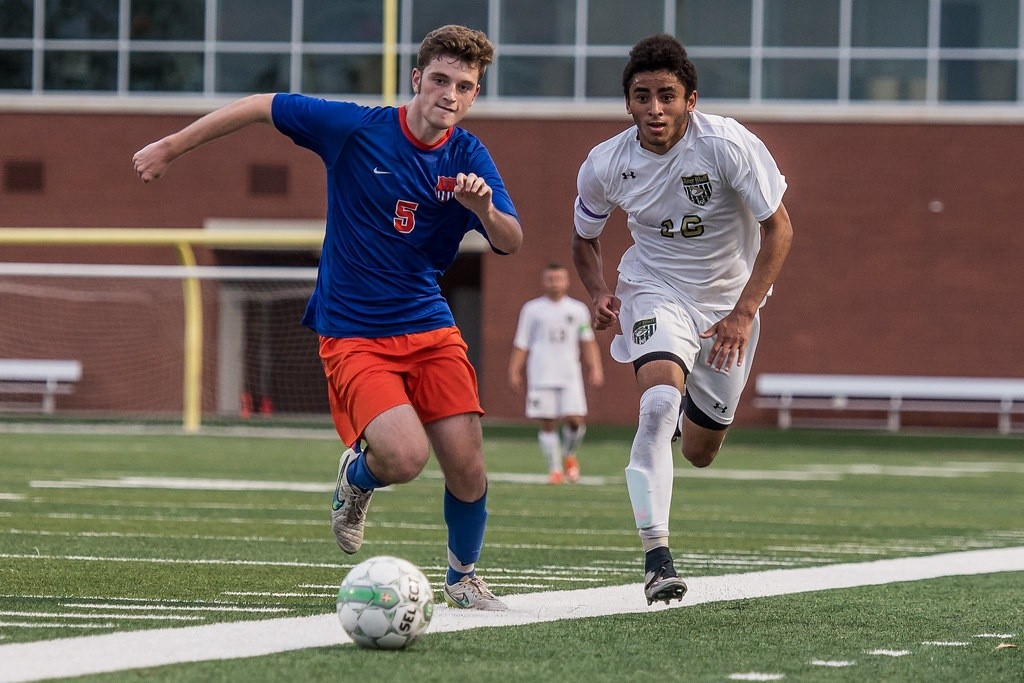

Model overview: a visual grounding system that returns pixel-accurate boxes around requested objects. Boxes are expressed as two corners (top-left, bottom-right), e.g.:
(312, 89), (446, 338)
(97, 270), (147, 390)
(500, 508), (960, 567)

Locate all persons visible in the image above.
(133, 25), (523, 613)
(507, 264), (602, 485)
(571, 32), (793, 606)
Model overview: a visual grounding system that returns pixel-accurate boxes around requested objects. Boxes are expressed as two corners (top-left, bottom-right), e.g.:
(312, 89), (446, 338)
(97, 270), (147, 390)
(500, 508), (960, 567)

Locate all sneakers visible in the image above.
(645, 547), (687, 606)
(330, 448), (375, 555)
(443, 571), (508, 610)
(671, 384), (687, 442)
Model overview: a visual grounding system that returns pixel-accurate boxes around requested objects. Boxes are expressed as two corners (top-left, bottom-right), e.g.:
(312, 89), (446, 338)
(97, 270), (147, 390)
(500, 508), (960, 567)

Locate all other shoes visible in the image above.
(547, 470), (562, 485)
(563, 457), (579, 482)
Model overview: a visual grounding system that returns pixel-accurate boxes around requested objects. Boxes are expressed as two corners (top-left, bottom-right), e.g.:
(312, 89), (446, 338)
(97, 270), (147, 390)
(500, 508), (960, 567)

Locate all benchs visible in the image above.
(0, 358), (84, 412)
(752, 373), (1024, 439)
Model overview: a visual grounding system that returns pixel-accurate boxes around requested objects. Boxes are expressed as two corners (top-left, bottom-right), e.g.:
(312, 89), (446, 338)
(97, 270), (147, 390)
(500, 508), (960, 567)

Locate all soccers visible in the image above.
(338, 553), (433, 653)
